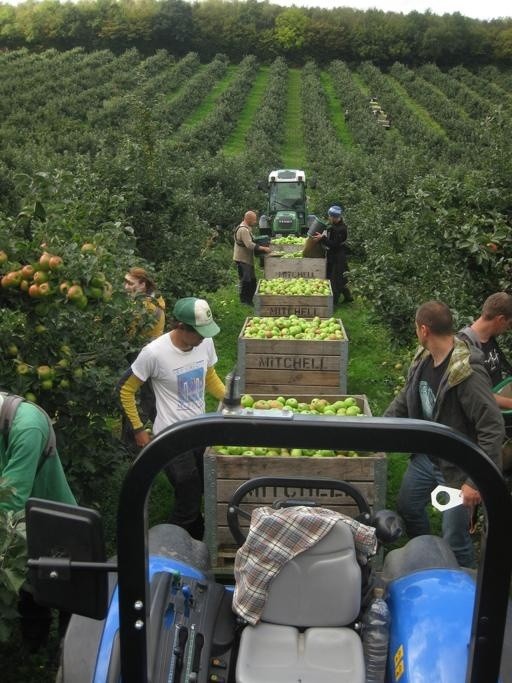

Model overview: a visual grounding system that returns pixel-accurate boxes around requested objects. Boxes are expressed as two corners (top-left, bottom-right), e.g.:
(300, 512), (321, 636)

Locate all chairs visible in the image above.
(234, 507), (365, 682)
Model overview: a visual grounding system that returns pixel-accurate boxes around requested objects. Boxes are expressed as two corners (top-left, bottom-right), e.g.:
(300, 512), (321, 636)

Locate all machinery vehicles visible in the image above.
(25, 413), (512, 683)
(257, 169), (317, 235)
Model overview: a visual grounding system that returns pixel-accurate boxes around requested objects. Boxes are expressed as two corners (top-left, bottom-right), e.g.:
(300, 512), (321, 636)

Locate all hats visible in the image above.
(172, 296), (221, 338)
(327, 206), (342, 216)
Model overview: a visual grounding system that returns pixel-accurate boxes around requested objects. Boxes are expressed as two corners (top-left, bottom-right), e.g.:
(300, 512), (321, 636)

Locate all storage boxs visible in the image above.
(263, 252), (327, 279)
(252, 279), (334, 318)
(237, 317), (349, 394)
(269, 240), (303, 251)
(203, 395), (388, 577)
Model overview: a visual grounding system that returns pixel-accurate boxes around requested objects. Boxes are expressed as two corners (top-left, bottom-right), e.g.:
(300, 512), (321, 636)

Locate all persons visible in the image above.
(121, 265), (165, 445)
(113, 297), (226, 543)
(344, 109), (350, 124)
(258, 212), (272, 238)
(0, 391), (79, 674)
(311, 205), (353, 303)
(232, 210), (271, 310)
(455, 291), (512, 537)
(381, 299), (505, 569)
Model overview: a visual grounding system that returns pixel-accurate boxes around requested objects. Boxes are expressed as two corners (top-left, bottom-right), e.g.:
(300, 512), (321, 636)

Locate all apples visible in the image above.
(391, 362), (407, 397)
(272, 250), (307, 261)
(257, 276), (332, 297)
(214, 394), (369, 460)
(0, 242), (114, 403)
(242, 314), (346, 343)
(271, 234), (307, 245)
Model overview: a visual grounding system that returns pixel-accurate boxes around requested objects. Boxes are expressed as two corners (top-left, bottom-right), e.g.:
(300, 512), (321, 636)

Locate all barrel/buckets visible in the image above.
(253, 234), (271, 258)
(492, 376), (512, 442)
(307, 216), (328, 240)
